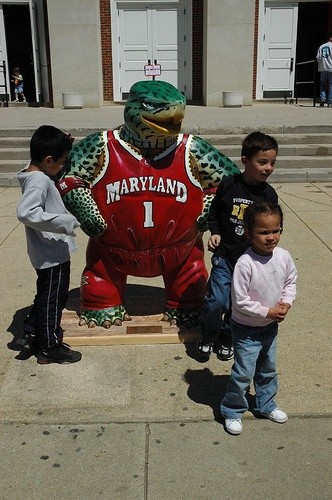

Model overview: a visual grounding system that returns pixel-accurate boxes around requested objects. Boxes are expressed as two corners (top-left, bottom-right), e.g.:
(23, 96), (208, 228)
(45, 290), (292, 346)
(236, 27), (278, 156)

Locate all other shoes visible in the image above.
(13, 98), (19, 102)
(22, 97), (26, 102)
(327, 99), (332, 103)
(24, 326), (36, 344)
(321, 98), (325, 105)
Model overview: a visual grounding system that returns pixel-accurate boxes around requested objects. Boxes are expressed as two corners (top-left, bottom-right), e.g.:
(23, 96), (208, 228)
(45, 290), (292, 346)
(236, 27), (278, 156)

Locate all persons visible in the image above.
(11, 67), (26, 102)
(219, 202), (297, 435)
(316, 33), (332, 107)
(197, 131), (283, 358)
(16, 125), (84, 365)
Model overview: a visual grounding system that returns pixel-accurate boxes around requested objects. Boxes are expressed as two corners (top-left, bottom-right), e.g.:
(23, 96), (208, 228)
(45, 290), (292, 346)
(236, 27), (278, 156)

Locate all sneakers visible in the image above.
(224, 416), (242, 435)
(197, 327), (214, 356)
(217, 343), (234, 361)
(37, 342), (81, 364)
(258, 404), (288, 424)
